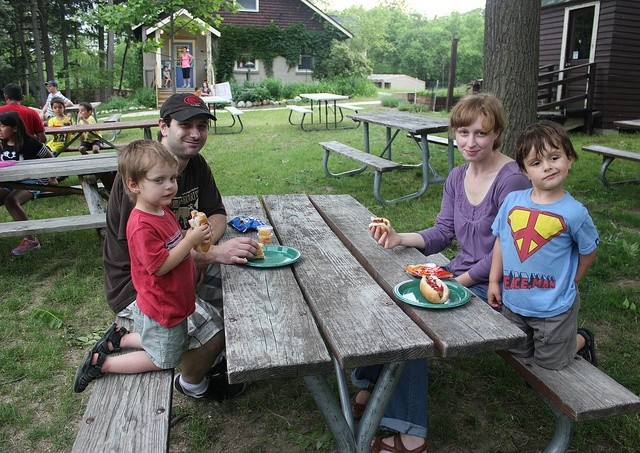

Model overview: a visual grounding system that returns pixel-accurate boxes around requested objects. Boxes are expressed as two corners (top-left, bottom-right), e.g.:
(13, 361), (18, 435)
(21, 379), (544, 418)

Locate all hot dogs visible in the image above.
(188, 209), (212, 253)
(254, 243), (265, 259)
(420, 275), (449, 304)
(368, 218), (391, 235)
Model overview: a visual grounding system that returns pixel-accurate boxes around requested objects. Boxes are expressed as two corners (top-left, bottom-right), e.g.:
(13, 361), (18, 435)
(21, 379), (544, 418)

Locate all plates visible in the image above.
(243, 244), (301, 267)
(392, 278), (472, 309)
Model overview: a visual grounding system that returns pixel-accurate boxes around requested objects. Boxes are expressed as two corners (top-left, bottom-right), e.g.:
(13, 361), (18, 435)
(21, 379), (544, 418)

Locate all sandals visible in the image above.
(371, 431), (427, 453)
(350, 387), (372, 423)
(92, 323), (129, 355)
(577, 328), (597, 368)
(74, 351), (106, 393)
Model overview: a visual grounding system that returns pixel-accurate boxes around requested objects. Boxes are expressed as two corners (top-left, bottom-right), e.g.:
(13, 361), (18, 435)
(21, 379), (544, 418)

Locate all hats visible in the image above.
(160, 92), (217, 122)
(44, 80), (57, 86)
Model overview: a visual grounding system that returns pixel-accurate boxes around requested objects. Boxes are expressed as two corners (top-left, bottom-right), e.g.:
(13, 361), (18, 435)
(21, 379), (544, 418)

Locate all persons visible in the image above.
(100, 92), (261, 402)
(44, 95), (78, 155)
(72, 137), (215, 395)
(39, 79), (74, 126)
(179, 46), (195, 88)
(486, 119), (602, 373)
(194, 86), (202, 97)
(200, 80), (213, 96)
(0, 82), (47, 144)
(66, 101), (104, 155)
(345, 88), (534, 452)
(0, 109), (60, 259)
(163, 65), (172, 89)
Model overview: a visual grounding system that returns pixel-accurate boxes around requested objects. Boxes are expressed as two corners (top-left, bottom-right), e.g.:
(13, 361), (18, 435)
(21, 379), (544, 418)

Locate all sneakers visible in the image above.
(11, 237), (41, 256)
(208, 355), (227, 377)
(174, 372), (245, 402)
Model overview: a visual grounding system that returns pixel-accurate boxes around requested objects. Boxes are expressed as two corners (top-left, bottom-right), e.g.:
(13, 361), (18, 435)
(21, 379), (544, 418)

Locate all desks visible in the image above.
(61, 101), (97, 125)
(43, 118), (158, 142)
(217, 194), (530, 452)
(614, 119), (640, 131)
(0, 150), (118, 206)
(344, 108), (456, 188)
(297, 91), (350, 128)
(197, 94), (231, 134)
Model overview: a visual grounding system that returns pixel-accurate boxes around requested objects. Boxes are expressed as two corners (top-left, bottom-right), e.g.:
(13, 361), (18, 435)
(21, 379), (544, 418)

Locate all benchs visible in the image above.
(103, 112), (123, 142)
(62, 142), (132, 153)
(331, 103), (363, 129)
(426, 251), (639, 453)
(223, 105), (244, 134)
(286, 102), (314, 132)
(581, 143), (640, 186)
(68, 314), (172, 453)
(406, 129), (458, 184)
(0, 211), (115, 239)
(317, 139), (426, 204)
(32, 182), (111, 198)
